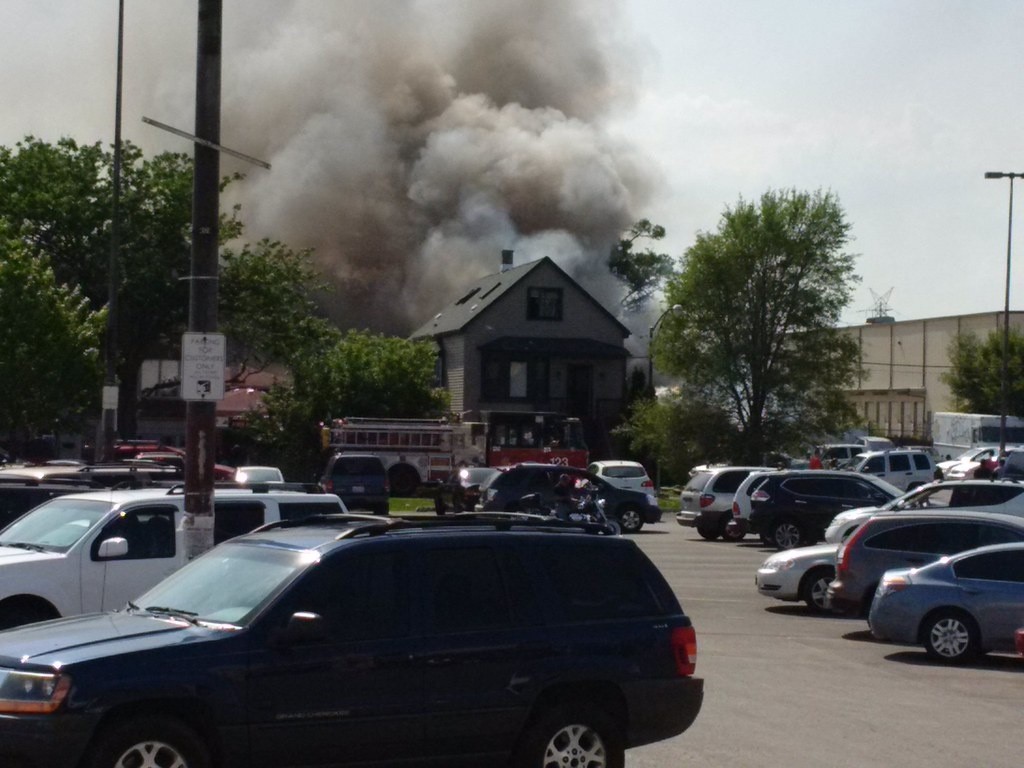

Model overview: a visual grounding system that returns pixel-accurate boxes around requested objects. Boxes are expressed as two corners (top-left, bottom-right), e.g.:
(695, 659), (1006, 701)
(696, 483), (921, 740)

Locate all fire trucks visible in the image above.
(320, 410), (590, 497)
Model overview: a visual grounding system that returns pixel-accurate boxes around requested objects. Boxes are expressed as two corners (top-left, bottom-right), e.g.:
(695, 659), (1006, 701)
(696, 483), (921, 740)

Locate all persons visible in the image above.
(974, 459), (1006, 480)
(550, 473), (573, 520)
(808, 449), (823, 469)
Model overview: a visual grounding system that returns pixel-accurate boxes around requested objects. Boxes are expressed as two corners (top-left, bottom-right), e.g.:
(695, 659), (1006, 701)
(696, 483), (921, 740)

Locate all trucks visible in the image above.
(932, 411), (1024, 460)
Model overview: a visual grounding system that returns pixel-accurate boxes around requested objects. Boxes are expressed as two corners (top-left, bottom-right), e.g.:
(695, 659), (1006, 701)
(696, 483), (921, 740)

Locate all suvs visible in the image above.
(827, 507), (1024, 617)
(0, 511), (705, 768)
(318, 449), (390, 516)
(676, 436), (1024, 543)
(0, 440), (349, 630)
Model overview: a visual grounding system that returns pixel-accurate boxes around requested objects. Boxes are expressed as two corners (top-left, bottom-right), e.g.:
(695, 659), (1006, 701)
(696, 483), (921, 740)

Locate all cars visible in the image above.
(755, 544), (847, 617)
(435, 458), (663, 533)
(868, 542), (1024, 665)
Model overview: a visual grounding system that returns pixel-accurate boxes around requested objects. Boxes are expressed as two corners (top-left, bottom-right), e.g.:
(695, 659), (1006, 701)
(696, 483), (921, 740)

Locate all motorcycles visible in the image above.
(519, 486), (621, 535)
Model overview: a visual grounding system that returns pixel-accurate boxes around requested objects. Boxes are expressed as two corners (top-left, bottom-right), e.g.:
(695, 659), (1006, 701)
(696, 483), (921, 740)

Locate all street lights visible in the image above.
(649, 304), (683, 401)
(985, 172), (1024, 453)
